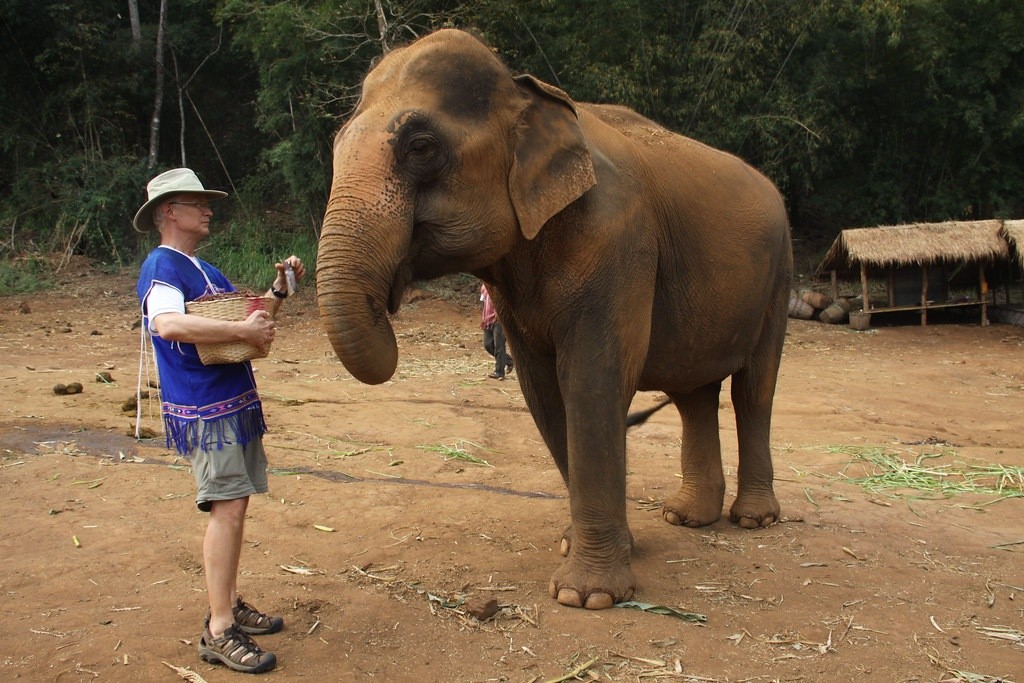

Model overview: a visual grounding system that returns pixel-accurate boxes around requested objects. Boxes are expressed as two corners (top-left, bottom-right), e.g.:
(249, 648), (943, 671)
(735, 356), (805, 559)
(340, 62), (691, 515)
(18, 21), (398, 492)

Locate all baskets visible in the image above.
(184, 296), (280, 365)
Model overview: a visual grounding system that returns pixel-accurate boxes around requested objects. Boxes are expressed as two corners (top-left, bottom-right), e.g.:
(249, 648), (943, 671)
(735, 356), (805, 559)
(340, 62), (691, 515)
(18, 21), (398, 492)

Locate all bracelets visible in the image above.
(270, 285), (289, 298)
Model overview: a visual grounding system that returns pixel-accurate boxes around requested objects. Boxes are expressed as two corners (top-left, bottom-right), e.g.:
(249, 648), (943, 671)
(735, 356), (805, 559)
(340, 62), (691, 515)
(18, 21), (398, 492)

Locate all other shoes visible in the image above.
(506, 364), (513, 374)
(488, 372), (504, 378)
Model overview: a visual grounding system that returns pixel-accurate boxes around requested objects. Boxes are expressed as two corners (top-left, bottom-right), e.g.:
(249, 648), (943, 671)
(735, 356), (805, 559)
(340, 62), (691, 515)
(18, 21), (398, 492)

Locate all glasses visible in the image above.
(169, 199), (211, 211)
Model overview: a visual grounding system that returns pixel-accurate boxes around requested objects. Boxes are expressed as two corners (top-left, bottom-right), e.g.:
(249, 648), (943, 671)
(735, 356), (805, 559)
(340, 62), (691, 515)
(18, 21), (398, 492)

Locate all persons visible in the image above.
(134, 166), (308, 671)
(478, 284), (512, 381)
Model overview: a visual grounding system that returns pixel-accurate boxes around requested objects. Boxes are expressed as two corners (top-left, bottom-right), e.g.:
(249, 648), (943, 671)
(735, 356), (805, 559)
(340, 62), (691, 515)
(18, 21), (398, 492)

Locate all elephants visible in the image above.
(315, 26), (792, 612)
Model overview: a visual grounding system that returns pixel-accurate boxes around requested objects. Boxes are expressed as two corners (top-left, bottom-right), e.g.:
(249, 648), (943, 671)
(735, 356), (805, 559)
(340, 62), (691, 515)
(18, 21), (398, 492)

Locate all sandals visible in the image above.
(198, 618), (276, 673)
(208, 594), (283, 633)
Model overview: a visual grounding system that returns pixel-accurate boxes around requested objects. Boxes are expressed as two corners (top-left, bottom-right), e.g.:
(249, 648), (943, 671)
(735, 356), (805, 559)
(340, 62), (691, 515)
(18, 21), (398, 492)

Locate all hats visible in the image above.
(133, 168), (228, 233)
